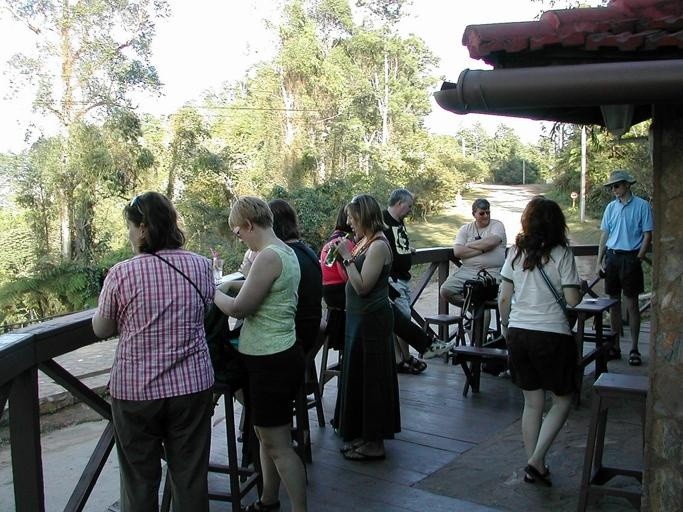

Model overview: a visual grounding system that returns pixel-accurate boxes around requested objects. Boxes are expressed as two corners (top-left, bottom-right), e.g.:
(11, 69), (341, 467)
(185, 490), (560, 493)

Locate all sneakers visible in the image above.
(422, 337), (456, 360)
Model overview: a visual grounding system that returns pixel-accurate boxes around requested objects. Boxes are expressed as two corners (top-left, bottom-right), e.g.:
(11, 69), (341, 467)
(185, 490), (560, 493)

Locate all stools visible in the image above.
(577, 373), (651, 512)
(160, 383), (262, 512)
(290, 358), (326, 431)
(465, 299), (501, 346)
(318, 332), (343, 398)
(418, 313), (463, 365)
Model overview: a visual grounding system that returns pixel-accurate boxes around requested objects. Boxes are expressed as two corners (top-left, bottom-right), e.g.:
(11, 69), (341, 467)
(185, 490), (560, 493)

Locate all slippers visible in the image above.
(339, 442), (359, 453)
(343, 448), (386, 461)
(527, 465), (552, 487)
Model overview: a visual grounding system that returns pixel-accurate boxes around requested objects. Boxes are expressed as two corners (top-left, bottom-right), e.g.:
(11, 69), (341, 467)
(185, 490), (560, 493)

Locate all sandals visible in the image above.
(397, 362), (420, 375)
(628, 349), (642, 366)
(408, 355), (427, 372)
(241, 498), (282, 512)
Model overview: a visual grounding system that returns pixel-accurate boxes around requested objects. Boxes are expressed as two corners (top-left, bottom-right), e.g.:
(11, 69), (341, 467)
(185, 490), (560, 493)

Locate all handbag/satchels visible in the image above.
(460, 267), (499, 321)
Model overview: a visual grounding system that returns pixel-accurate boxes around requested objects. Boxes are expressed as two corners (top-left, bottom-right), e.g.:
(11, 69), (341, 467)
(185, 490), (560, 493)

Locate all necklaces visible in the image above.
(352, 230), (376, 256)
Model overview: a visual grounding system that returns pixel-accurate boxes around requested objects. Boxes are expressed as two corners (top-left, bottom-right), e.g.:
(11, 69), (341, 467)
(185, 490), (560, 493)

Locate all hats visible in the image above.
(603, 169), (637, 187)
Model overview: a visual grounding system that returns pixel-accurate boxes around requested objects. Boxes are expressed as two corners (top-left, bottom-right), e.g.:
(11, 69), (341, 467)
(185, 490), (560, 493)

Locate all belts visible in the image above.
(608, 248), (637, 255)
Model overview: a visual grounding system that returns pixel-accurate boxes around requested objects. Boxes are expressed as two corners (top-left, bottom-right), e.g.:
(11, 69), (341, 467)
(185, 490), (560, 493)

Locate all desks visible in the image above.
(564, 297), (620, 377)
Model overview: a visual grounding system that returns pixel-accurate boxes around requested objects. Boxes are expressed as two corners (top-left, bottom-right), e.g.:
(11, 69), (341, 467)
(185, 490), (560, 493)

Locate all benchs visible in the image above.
(453, 345), (509, 398)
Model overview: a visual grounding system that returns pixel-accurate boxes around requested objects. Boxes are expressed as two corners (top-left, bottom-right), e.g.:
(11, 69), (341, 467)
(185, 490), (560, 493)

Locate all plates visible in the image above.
(582, 299), (598, 303)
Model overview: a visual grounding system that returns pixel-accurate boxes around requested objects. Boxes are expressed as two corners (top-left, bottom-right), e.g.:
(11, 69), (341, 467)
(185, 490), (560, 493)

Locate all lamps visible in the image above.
(600, 97), (648, 146)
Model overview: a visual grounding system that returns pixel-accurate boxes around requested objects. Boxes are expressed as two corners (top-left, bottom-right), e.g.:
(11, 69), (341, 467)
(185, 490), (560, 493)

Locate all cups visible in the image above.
(323, 243), (339, 268)
(213, 259), (224, 285)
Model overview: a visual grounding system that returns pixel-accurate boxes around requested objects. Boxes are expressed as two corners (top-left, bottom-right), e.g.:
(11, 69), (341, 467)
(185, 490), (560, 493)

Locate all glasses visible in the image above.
(233, 225), (241, 237)
(478, 210), (490, 215)
(130, 194), (145, 215)
(610, 181), (625, 190)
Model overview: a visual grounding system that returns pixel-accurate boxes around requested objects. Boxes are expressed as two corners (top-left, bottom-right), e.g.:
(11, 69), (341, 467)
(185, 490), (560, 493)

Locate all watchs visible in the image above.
(343, 257), (355, 267)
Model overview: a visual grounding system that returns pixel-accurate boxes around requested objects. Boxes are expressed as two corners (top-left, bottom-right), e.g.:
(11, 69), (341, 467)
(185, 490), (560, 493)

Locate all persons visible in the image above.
(92, 192), (216, 512)
(595, 169), (654, 366)
(238, 200), (323, 366)
(321, 205), (455, 357)
(440, 199), (507, 315)
(214, 197), (308, 512)
(499, 195), (589, 488)
(382, 188), (427, 374)
